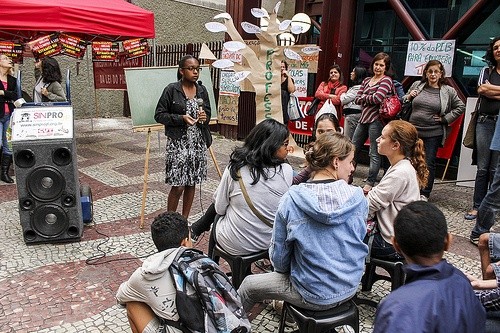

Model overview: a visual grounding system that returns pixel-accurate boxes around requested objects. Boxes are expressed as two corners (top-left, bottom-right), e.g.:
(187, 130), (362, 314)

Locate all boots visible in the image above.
(1, 153), (14, 183)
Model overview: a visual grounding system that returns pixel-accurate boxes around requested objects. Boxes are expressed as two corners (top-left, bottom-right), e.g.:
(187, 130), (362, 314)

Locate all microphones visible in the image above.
(197, 97), (204, 125)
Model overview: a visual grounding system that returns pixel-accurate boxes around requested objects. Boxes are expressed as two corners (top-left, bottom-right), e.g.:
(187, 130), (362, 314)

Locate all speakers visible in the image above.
(11, 137), (83, 246)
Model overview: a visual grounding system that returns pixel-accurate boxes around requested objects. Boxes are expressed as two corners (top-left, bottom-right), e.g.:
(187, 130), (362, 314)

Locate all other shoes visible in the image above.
(187, 225), (199, 243)
(254, 257), (274, 273)
(464, 209), (478, 220)
(470, 236), (479, 245)
(362, 184), (373, 194)
(421, 195), (428, 202)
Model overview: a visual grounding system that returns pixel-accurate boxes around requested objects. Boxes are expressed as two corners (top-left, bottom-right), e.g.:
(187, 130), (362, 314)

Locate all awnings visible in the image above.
(0, 0), (157, 130)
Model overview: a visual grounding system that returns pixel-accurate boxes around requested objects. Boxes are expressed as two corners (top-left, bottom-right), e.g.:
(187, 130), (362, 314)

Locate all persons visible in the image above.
(0, 54), (18, 183)
(372, 201), (486, 333)
(299, 52), (407, 195)
(188, 118), (293, 265)
(236, 132), (369, 326)
(32, 56), (67, 102)
(401, 60), (466, 203)
(281, 60), (297, 127)
(154, 55), (212, 222)
(116, 211), (209, 333)
(366, 120), (431, 262)
(293, 113), (357, 185)
(464, 36), (500, 318)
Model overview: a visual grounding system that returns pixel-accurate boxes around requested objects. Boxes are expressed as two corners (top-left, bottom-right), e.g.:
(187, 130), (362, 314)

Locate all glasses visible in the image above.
(0, 56), (13, 61)
(428, 69), (441, 73)
(181, 66), (202, 72)
(281, 140), (289, 147)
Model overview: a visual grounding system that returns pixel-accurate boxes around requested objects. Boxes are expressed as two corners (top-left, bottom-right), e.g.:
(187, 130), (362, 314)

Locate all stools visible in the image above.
(210, 241), (273, 289)
(278, 298), (360, 333)
(361, 256), (407, 293)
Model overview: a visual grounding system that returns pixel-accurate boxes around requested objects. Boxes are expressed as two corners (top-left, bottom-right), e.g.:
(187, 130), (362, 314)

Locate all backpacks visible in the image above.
(158, 248), (252, 333)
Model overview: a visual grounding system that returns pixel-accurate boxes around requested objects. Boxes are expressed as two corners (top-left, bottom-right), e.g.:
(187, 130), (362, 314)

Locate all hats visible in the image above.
(314, 98), (338, 124)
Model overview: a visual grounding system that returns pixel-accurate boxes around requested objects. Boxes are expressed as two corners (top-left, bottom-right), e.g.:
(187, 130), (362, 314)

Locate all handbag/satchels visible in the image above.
(396, 81), (423, 122)
(287, 92), (305, 121)
(364, 213), (377, 236)
(306, 80), (328, 116)
(377, 76), (402, 118)
(463, 66), (492, 149)
